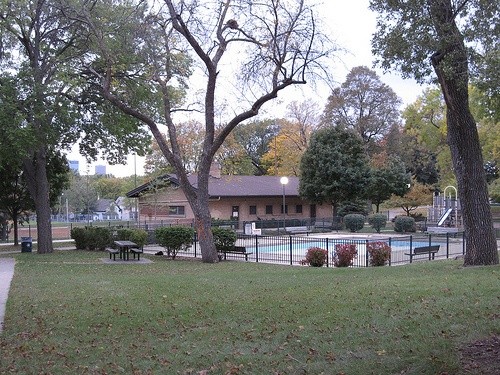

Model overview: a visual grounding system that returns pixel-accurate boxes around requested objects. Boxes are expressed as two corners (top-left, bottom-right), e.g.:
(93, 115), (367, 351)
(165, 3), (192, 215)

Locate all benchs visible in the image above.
(216, 245), (253, 261)
(404, 244), (440, 265)
(105, 248), (119, 258)
(132, 248), (143, 260)
(285, 225), (313, 235)
(427, 226), (459, 237)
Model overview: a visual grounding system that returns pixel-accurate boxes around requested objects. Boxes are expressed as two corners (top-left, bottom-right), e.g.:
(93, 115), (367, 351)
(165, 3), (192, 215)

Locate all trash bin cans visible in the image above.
(21, 237), (33, 252)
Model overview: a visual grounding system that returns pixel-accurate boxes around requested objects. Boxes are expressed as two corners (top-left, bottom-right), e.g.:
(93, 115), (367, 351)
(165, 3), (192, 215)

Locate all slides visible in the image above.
(436, 207), (452, 226)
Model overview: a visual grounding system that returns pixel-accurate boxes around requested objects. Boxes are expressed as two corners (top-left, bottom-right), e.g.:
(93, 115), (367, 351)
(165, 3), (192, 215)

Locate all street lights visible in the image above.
(280, 177), (289, 230)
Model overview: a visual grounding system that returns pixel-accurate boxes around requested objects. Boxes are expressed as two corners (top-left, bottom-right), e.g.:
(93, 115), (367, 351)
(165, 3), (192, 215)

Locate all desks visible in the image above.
(114, 241), (137, 260)
